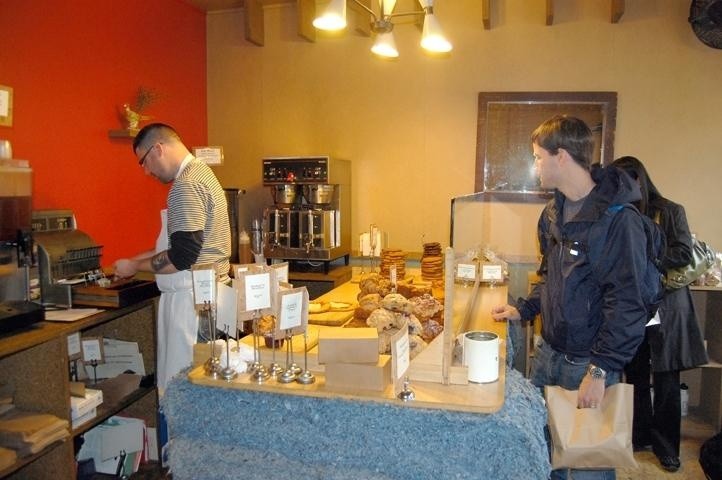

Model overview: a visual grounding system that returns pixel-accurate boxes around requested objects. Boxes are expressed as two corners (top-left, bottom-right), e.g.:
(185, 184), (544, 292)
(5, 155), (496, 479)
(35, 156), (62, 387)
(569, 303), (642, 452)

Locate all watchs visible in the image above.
(586, 363), (605, 382)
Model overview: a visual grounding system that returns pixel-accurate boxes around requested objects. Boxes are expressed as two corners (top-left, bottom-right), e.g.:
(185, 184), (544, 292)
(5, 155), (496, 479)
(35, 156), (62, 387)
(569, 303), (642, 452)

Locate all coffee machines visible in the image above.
(0, 158), (45, 336)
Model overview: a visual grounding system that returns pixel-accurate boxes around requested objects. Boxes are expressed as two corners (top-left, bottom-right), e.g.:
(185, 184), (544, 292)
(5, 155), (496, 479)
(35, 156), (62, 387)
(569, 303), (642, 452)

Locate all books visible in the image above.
(75, 410), (160, 477)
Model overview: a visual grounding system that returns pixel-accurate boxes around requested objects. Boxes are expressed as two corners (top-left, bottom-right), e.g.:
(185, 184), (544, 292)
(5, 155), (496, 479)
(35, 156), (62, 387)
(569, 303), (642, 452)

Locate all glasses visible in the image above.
(139, 143), (163, 167)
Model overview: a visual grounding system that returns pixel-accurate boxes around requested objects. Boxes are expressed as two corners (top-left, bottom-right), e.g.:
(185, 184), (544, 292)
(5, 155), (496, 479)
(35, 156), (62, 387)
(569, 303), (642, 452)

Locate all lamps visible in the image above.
(314, 0), (452, 57)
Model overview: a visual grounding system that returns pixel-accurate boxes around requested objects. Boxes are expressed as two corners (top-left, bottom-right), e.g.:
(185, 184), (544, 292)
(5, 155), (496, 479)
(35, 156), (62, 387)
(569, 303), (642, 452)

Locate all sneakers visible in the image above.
(660, 456), (680, 472)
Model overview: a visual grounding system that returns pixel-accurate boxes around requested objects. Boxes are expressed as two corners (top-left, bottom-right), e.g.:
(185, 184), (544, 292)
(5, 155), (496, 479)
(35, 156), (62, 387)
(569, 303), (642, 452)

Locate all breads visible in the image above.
(354, 242), (444, 359)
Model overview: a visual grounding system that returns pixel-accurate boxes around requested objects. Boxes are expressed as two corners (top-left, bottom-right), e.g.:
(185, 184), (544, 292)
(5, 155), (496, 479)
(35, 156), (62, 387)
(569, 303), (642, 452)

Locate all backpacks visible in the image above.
(540, 202), (668, 325)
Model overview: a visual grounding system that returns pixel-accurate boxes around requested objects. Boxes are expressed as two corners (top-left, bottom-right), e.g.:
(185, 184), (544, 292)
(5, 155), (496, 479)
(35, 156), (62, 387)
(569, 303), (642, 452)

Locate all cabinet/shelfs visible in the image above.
(0, 298), (159, 480)
(677, 285), (721, 438)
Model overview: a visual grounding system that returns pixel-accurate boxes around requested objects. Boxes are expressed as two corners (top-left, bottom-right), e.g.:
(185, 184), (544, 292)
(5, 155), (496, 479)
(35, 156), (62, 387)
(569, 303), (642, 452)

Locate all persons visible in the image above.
(606, 153), (709, 472)
(109, 121), (234, 408)
(487, 113), (651, 480)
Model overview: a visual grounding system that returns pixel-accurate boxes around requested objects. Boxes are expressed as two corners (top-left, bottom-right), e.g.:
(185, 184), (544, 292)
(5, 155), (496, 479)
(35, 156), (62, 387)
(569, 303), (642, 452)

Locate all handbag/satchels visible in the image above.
(660, 241), (716, 290)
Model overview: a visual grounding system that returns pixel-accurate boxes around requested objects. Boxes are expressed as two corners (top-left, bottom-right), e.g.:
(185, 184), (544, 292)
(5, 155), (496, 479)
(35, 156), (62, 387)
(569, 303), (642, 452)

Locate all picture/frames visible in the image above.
(475, 89), (617, 206)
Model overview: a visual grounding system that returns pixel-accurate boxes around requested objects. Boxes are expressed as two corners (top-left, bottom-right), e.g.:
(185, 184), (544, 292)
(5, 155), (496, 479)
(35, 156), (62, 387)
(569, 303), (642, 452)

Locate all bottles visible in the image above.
(680, 382), (689, 418)
(238, 227), (252, 264)
